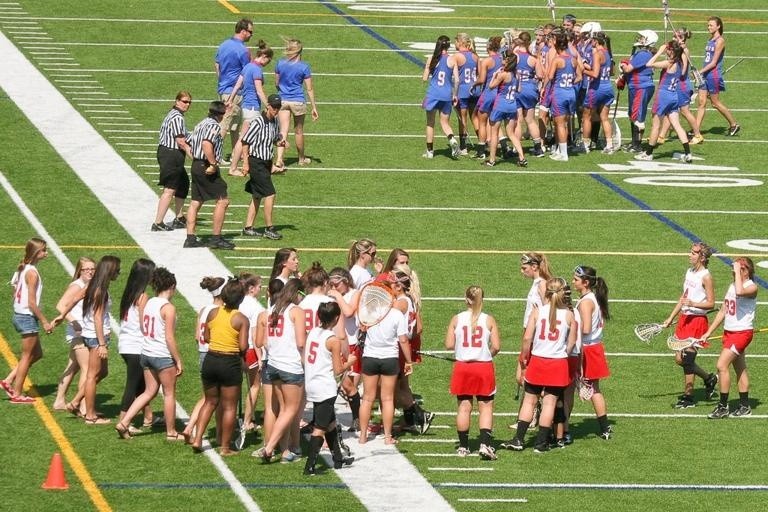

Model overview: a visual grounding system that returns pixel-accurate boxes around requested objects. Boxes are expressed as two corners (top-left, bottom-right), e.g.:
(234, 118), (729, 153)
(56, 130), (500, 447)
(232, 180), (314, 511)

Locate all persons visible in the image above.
(697, 255), (759, 419)
(615, 16), (740, 164)
(184, 237), (436, 475)
(2, 237), (190, 442)
(422, 15), (615, 167)
(446, 250), (614, 461)
(150, 17), (320, 250)
(660, 241), (718, 411)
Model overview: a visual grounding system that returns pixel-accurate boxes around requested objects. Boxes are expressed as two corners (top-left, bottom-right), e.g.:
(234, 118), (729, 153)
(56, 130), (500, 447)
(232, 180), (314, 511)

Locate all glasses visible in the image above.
(81, 268), (96, 273)
(522, 254), (538, 264)
(329, 275), (349, 285)
(365, 251), (376, 257)
(271, 105), (280, 109)
(179, 99), (191, 104)
(246, 30), (252, 34)
(268, 95), (281, 105)
(574, 265), (595, 279)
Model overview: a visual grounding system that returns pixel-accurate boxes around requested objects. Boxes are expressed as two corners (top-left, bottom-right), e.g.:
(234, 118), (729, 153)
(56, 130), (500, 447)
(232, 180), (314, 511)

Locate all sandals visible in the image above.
(166, 433), (185, 440)
(67, 402), (80, 413)
(85, 417), (112, 425)
(115, 422), (133, 440)
(219, 447), (239, 456)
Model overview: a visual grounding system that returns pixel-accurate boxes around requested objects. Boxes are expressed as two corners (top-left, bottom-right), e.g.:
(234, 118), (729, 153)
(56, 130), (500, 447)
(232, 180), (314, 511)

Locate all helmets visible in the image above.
(580, 22), (601, 46)
(633, 30), (658, 48)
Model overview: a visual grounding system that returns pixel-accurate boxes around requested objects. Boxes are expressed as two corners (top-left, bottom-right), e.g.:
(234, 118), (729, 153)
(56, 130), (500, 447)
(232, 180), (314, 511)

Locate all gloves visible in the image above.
(618, 60), (629, 73)
(614, 73), (626, 90)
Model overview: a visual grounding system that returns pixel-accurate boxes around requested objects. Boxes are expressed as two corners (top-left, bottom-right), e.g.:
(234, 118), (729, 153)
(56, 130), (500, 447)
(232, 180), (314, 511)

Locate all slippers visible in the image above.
(143, 416), (165, 426)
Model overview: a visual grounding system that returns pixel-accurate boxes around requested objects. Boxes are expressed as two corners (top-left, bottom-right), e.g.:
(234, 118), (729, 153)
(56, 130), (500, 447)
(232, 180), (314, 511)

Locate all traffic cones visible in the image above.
(39, 453), (71, 490)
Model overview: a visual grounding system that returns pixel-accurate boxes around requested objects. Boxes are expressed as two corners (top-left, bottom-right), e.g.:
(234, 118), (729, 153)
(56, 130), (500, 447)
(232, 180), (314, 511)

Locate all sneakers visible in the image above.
(707, 402), (729, 419)
(422, 149), (434, 159)
(726, 124), (740, 136)
(533, 440), (551, 453)
(679, 154), (692, 163)
(400, 424), (417, 432)
(500, 437), (524, 451)
(279, 451), (302, 465)
(478, 442), (498, 461)
(276, 160), (283, 168)
(502, 151), (519, 158)
(647, 135), (665, 144)
(9, 395), (37, 403)
(449, 137), (461, 159)
(334, 457), (354, 469)
(634, 151), (653, 160)
(151, 221), (174, 231)
(704, 373), (719, 399)
(572, 142), (590, 152)
(184, 236), (207, 247)
(418, 410), (435, 435)
(377, 403), (405, 418)
(530, 148), (545, 158)
(264, 226), (283, 240)
(676, 395), (696, 408)
(0, 380), (15, 398)
(688, 133), (704, 145)
(290, 447), (303, 456)
(480, 160), (496, 167)
(472, 152), (486, 160)
(366, 420), (379, 435)
(456, 446), (471, 457)
(298, 158), (311, 166)
(621, 144), (643, 153)
(601, 146), (615, 154)
(303, 466), (324, 476)
(208, 236), (235, 250)
(687, 129), (694, 136)
(258, 448), (271, 463)
(729, 404), (753, 417)
(509, 423), (536, 430)
(242, 226), (263, 238)
(550, 438), (565, 449)
(460, 147), (468, 155)
(172, 216), (187, 228)
(300, 420), (315, 433)
(347, 418), (361, 432)
(549, 151), (569, 161)
(511, 158), (528, 167)
(599, 425), (611, 440)
(549, 433), (573, 444)
